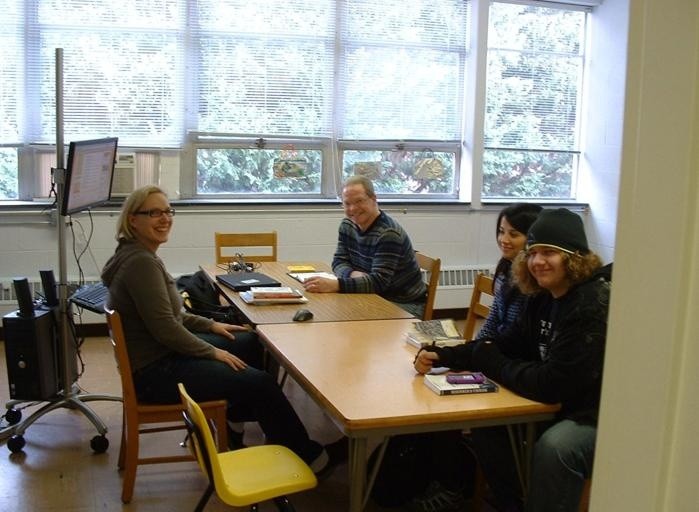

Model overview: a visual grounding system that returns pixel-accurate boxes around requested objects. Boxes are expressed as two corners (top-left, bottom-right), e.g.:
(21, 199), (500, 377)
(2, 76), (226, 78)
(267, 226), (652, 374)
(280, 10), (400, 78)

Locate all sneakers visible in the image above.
(407, 480), (463, 511)
(226, 423), (247, 451)
(313, 436), (349, 481)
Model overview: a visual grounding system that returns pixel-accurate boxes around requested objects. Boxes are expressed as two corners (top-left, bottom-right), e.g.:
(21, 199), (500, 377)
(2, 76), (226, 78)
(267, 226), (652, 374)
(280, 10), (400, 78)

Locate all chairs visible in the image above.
(175, 381), (318, 512)
(215, 231), (278, 263)
(412, 250), (441, 321)
(463, 271), (495, 340)
(102, 301), (228, 501)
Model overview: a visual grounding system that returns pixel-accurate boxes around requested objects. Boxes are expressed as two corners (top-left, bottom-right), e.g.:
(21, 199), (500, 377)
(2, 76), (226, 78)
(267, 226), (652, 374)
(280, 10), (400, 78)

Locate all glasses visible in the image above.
(137, 208), (175, 217)
(342, 197), (369, 207)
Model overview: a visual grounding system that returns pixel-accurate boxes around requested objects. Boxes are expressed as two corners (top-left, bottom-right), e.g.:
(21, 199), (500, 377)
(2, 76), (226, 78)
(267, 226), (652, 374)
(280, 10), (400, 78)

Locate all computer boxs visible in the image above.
(2, 270), (80, 403)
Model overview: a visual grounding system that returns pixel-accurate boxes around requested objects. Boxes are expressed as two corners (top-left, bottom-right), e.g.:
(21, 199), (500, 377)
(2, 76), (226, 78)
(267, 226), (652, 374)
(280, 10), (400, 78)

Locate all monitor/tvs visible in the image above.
(61, 137), (118, 216)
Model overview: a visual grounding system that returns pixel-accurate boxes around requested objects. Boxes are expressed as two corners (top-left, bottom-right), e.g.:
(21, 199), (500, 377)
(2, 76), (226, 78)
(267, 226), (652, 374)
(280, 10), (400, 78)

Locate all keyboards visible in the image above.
(69, 282), (111, 314)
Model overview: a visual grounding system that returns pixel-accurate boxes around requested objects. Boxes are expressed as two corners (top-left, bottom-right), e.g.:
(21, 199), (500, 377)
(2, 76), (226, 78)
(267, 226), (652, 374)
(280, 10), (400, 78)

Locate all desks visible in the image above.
(253, 319), (564, 512)
(198, 260), (419, 324)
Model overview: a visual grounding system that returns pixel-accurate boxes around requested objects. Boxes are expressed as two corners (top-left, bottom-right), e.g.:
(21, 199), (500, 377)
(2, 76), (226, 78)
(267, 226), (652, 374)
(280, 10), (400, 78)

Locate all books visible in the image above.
(422, 370), (499, 397)
(238, 285), (309, 307)
(403, 316), (467, 349)
(286, 265), (316, 272)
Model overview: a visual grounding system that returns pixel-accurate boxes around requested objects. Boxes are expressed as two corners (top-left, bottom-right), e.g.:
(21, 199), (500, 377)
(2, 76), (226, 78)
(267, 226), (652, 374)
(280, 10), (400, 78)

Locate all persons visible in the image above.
(411, 202), (543, 509)
(302, 175), (429, 320)
(416, 206), (613, 512)
(101, 184), (351, 483)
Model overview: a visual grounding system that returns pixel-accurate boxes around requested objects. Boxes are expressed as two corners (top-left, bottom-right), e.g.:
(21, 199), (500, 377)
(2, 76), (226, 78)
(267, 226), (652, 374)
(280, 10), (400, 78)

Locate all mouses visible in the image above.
(293, 309), (314, 321)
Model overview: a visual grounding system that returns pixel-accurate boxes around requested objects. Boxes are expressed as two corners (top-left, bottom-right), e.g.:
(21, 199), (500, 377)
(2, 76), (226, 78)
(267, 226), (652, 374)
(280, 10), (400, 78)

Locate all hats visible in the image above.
(525, 208), (588, 258)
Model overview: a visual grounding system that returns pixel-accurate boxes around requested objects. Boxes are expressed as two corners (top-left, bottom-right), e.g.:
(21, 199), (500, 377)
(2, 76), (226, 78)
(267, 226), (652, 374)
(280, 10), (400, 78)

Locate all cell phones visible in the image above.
(446, 373), (485, 384)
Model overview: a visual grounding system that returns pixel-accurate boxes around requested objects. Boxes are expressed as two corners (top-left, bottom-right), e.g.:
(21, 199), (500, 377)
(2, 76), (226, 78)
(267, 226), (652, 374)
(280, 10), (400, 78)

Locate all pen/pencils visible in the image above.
(432, 341), (435, 346)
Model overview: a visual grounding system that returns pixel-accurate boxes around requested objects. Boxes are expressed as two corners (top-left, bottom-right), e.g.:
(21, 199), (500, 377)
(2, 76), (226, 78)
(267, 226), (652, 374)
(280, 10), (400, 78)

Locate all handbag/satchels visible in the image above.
(177, 270), (221, 310)
(213, 306), (240, 325)
(367, 429), (477, 507)
